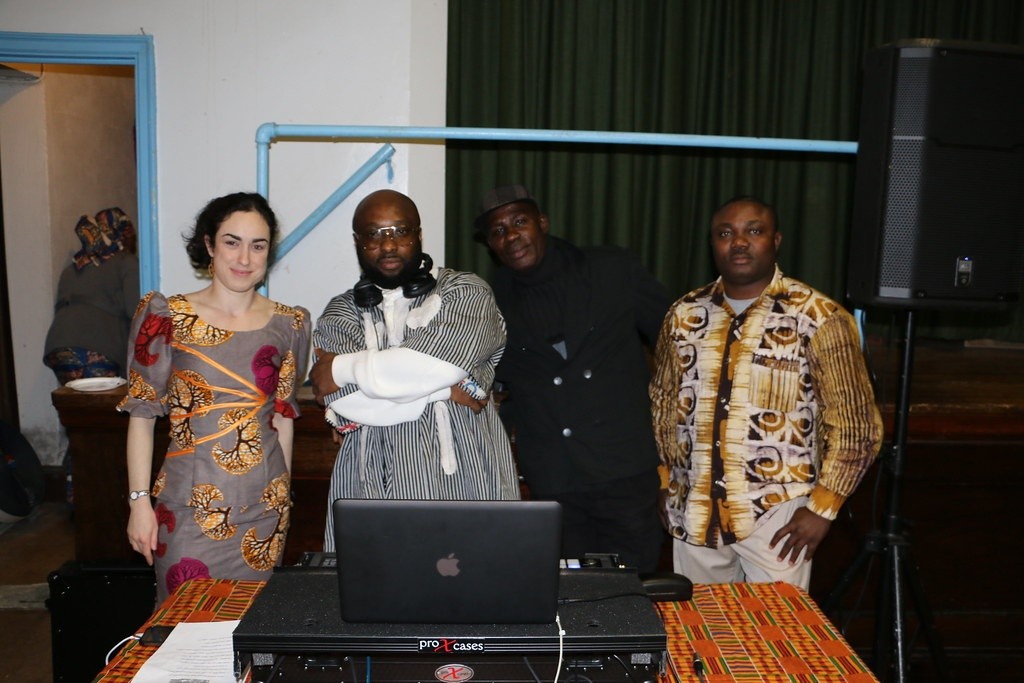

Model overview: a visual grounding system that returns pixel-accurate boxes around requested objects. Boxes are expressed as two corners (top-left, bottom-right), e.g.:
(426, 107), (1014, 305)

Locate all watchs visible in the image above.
(128, 490), (151, 499)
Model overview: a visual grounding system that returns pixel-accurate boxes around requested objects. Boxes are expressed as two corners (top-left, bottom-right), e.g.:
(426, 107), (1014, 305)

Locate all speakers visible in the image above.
(847, 37), (1024, 318)
(48, 558), (158, 683)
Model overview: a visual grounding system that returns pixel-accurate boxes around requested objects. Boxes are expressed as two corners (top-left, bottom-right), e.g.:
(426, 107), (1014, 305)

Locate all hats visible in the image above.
(481, 184), (529, 214)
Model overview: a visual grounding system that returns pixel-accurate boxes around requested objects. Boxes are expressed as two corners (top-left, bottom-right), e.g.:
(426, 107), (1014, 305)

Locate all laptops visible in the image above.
(332, 498), (563, 623)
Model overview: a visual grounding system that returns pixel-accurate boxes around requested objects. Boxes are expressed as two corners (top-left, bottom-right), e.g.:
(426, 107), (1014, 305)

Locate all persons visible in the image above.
(42, 207), (142, 507)
(309, 191), (520, 552)
(473, 185), (663, 573)
(117, 192), (312, 612)
(650, 197), (882, 593)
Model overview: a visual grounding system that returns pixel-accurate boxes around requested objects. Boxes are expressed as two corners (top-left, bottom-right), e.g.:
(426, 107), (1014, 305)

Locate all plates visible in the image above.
(65, 376), (126, 392)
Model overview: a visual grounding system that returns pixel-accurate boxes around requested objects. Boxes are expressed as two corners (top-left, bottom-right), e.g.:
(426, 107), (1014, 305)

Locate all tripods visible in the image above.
(818, 310), (950, 683)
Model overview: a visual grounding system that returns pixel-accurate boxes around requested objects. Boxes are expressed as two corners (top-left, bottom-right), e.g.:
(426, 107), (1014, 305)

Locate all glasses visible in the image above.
(353, 226), (421, 242)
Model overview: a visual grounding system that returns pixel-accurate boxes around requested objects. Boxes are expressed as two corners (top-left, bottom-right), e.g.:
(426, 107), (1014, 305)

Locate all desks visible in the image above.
(89, 576), (882, 683)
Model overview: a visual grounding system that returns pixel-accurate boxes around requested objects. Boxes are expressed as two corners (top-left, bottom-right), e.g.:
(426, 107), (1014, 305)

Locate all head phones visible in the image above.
(354, 253), (436, 308)
